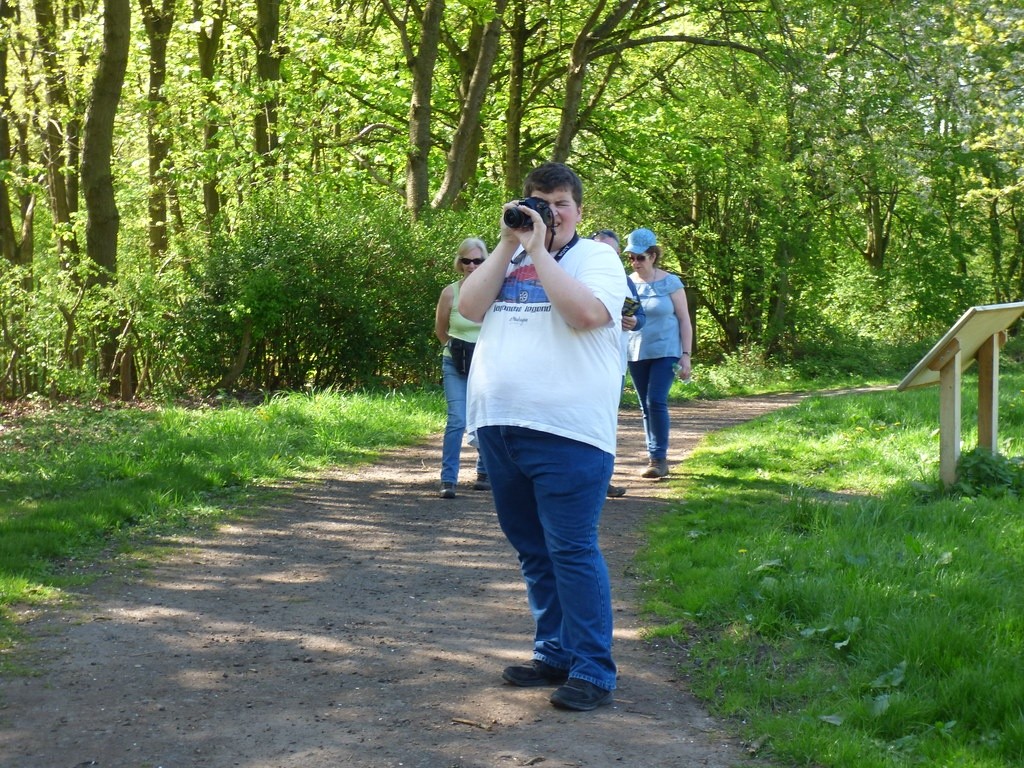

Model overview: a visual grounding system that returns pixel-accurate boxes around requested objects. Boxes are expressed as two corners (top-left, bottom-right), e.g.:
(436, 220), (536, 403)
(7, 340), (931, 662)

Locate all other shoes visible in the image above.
(642, 455), (669, 478)
(604, 481), (626, 497)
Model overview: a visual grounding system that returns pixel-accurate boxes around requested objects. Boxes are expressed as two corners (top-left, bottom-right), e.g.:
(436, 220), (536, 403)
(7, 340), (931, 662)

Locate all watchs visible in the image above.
(683, 352), (691, 356)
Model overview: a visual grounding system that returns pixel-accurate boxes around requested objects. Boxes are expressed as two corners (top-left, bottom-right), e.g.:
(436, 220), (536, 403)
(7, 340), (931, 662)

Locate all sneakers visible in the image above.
(502, 657), (568, 686)
(550, 677), (612, 711)
(438, 480), (456, 498)
(473, 473), (494, 491)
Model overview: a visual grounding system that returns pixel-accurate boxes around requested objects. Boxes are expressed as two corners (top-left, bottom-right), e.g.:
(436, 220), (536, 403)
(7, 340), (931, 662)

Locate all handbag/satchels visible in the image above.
(448, 336), (477, 375)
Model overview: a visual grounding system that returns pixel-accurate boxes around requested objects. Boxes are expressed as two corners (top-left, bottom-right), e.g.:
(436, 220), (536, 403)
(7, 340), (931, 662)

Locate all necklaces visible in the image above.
(643, 269), (657, 308)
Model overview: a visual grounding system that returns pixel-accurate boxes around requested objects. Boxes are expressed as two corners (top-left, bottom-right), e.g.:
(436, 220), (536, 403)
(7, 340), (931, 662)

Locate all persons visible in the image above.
(590, 230), (646, 495)
(436, 238), (493, 498)
(623, 229), (692, 478)
(457, 162), (627, 710)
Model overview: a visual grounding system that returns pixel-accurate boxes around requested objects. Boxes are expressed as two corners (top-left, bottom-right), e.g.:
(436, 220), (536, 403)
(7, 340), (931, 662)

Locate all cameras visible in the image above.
(504, 196), (554, 230)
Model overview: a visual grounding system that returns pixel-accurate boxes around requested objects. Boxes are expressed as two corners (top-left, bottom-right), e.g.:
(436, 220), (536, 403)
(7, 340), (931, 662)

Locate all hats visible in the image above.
(623, 229), (657, 255)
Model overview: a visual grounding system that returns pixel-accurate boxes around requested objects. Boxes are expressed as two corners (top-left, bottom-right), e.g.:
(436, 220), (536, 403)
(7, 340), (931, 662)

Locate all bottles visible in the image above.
(673, 363), (691, 385)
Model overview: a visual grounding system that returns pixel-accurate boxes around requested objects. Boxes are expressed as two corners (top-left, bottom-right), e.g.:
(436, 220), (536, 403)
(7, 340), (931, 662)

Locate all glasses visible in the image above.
(628, 254), (651, 262)
(458, 258), (483, 266)
